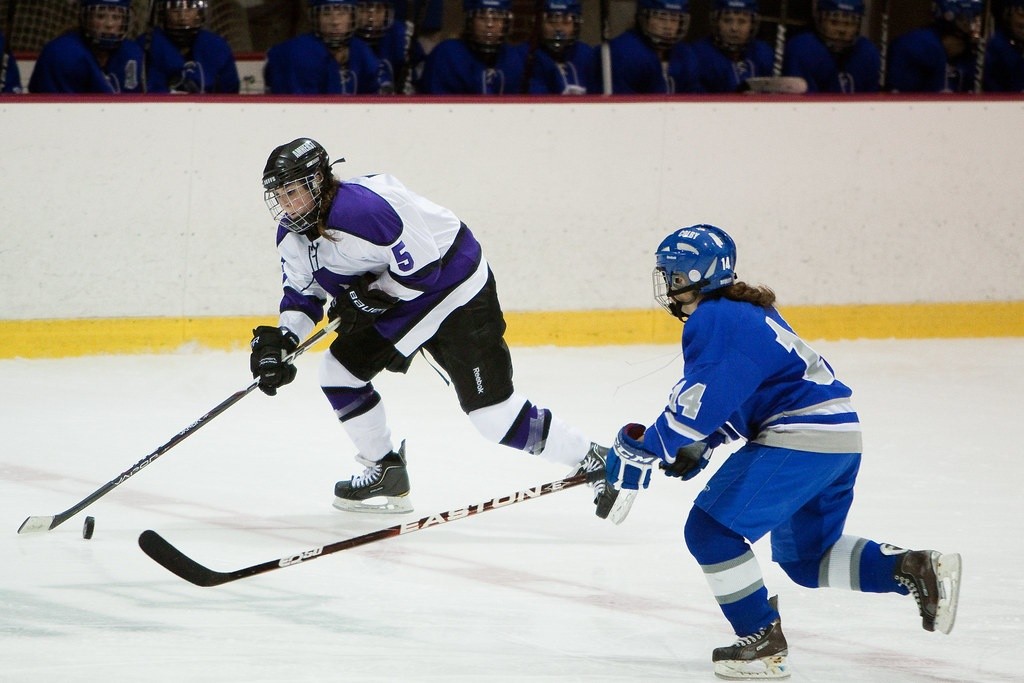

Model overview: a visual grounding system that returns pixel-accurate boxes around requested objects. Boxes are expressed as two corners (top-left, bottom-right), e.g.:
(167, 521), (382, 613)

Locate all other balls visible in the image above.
(83, 516), (95, 539)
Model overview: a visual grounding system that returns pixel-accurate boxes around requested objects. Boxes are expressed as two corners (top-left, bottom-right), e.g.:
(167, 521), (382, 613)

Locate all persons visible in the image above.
(250, 138), (640, 525)
(770, 0), (882, 94)
(417, 0), (592, 95)
(27, 0), (147, 95)
(582, 0), (782, 94)
(885, 0), (1024, 93)
(605, 225), (962, 682)
(262, 0), (427, 97)
(0, 32), (22, 94)
(135, 0), (239, 94)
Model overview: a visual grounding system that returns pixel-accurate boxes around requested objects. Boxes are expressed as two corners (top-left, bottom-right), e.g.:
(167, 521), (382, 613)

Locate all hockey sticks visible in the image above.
(879, 0), (889, 91)
(402, 0), (416, 94)
(16, 317), (341, 533)
(138, 468), (607, 587)
(772, 0), (786, 77)
(975, 0), (990, 92)
(600, 0), (613, 94)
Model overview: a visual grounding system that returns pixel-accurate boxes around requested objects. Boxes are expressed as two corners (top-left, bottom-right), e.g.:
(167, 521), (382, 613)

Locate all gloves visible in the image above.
(250, 326), (298, 396)
(659, 433), (726, 482)
(606, 423), (657, 490)
(327, 271), (399, 335)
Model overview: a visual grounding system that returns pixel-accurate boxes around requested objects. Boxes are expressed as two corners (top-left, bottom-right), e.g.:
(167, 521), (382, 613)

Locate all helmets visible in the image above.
(933, 0), (982, 44)
(652, 225), (737, 316)
(78, 0), (131, 50)
(353, 0), (395, 38)
(537, 0), (581, 53)
(636, 0), (691, 47)
(307, 0), (357, 48)
(157, 0), (207, 43)
(710, 0), (762, 53)
(463, 0), (516, 54)
(817, 0), (862, 47)
(262, 138), (330, 235)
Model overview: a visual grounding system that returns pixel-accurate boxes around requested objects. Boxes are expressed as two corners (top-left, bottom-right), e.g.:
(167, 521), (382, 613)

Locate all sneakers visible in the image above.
(334, 439), (413, 514)
(880, 543), (960, 635)
(712, 595), (790, 678)
(579, 442), (636, 522)
